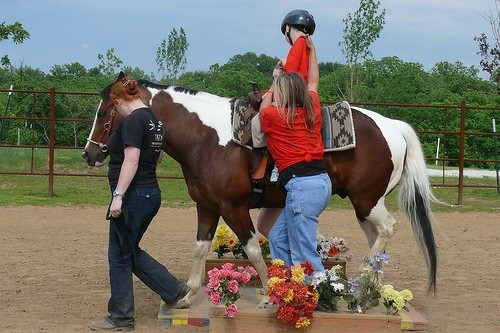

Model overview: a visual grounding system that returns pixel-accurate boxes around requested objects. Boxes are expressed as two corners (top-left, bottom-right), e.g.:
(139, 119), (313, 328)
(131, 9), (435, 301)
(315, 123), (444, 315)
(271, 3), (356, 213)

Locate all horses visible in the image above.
(81, 70), (462, 307)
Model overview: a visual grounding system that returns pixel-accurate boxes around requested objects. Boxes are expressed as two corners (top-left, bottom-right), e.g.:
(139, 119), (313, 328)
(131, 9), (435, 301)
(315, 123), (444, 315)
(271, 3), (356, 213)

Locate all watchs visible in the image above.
(113, 191), (124, 197)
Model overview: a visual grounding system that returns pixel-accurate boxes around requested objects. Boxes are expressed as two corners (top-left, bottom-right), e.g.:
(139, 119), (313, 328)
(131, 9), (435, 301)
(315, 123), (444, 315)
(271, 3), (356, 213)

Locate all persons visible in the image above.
(270, 9), (316, 182)
(89, 76), (192, 333)
(259, 35), (332, 284)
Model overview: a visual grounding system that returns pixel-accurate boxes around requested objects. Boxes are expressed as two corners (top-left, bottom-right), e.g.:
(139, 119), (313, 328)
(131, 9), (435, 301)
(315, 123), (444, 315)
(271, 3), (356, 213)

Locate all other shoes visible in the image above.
(90, 319), (134, 331)
(270, 167), (279, 182)
(162, 285), (194, 314)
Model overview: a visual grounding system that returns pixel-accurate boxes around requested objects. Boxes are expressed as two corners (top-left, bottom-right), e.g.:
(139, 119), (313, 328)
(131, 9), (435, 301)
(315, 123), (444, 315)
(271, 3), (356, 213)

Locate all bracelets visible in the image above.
(268, 90), (273, 93)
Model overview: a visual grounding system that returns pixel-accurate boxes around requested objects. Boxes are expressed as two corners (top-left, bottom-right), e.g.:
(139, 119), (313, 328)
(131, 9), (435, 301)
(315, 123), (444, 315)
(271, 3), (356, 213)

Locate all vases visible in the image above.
(207, 293), (428, 332)
(206, 254), (346, 286)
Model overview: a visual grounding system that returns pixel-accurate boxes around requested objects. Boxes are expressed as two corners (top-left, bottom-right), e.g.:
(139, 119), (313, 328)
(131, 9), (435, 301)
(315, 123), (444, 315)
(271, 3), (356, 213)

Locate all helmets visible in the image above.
(281, 10), (315, 35)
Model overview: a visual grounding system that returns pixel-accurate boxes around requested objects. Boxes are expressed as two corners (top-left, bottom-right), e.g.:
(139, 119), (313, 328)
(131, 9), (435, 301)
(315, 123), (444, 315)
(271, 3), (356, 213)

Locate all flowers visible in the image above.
(210, 223), (352, 261)
(204, 262), (259, 318)
(261, 252), (413, 328)
